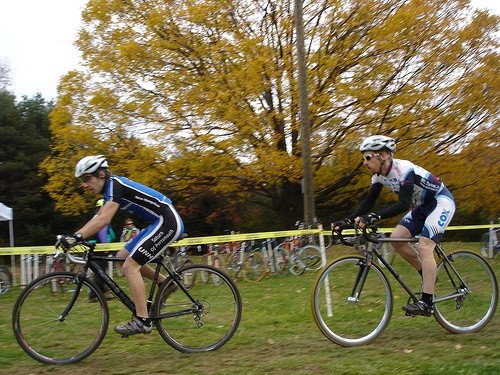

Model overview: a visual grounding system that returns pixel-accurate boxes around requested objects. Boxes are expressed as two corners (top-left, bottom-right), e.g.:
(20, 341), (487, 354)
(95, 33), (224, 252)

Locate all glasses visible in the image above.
(361, 153), (380, 161)
(126, 223), (134, 225)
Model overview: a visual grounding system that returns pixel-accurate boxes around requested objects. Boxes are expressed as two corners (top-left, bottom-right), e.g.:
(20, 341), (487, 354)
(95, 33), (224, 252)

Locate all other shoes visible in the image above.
(88, 293), (115, 303)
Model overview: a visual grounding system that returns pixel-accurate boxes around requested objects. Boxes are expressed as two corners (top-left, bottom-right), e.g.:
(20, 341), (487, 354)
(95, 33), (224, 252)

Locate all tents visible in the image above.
(0, 202), (17, 286)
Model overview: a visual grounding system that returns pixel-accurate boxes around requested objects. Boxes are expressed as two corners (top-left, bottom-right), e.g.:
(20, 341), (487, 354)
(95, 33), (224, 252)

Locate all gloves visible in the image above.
(55, 233), (83, 254)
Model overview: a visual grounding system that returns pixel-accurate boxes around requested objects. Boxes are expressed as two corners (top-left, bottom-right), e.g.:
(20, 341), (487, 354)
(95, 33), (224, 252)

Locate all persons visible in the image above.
(55, 156), (183, 335)
(331, 135), (456, 318)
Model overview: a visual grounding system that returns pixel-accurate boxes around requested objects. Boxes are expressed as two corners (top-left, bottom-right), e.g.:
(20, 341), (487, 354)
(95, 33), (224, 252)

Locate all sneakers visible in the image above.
(114, 316), (153, 337)
(402, 299), (435, 317)
(154, 277), (178, 304)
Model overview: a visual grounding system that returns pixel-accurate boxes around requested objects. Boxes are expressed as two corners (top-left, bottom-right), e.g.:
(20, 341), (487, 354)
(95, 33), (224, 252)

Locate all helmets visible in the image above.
(74, 154), (109, 178)
(359, 135), (397, 153)
(96, 198), (105, 207)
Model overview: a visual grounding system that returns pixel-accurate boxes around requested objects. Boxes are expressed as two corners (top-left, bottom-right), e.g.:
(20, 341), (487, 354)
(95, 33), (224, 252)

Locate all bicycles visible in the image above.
(170, 220), (333, 290)
(479, 226), (500, 262)
(10, 235), (243, 366)
(308, 215), (499, 349)
(0, 266), (13, 295)
